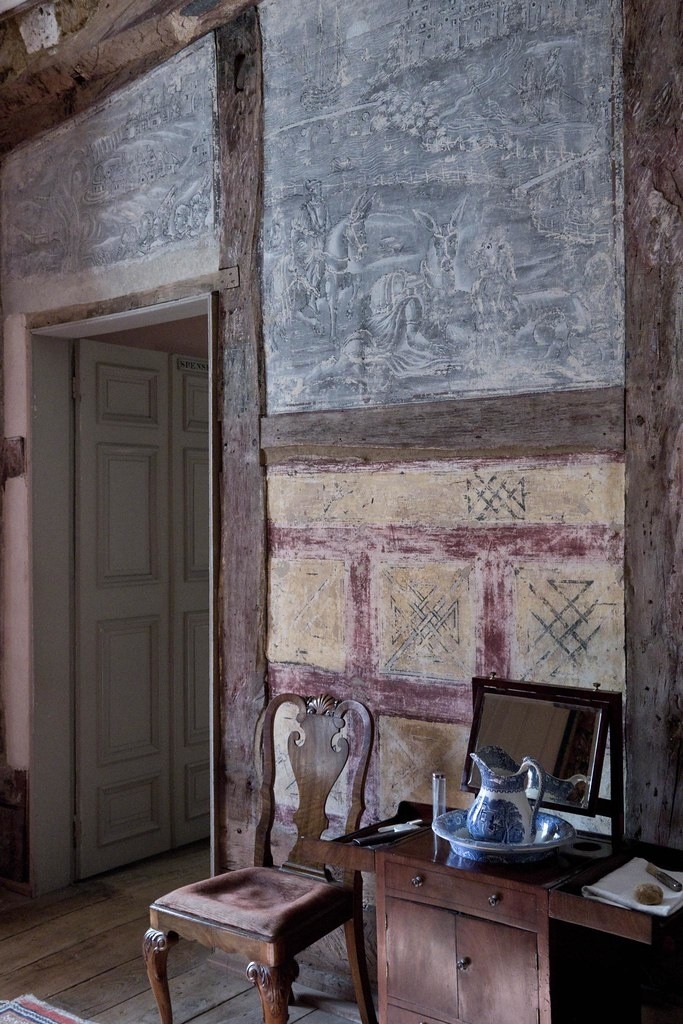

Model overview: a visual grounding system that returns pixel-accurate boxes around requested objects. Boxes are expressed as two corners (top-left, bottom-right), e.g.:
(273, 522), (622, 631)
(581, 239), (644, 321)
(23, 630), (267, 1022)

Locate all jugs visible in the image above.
(467, 744), (546, 845)
(523, 757), (590, 805)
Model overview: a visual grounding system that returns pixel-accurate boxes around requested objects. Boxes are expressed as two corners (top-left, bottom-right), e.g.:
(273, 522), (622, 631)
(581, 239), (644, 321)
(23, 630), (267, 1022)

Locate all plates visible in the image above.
(433, 811), (575, 866)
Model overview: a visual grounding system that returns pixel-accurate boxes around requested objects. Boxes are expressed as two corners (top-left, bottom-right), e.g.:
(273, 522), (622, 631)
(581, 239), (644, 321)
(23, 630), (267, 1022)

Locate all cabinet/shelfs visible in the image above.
(302, 801), (683, 1024)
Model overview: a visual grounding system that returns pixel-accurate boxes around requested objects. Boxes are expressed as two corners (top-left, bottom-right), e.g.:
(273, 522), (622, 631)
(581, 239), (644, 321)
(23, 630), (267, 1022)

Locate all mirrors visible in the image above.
(450, 672), (626, 835)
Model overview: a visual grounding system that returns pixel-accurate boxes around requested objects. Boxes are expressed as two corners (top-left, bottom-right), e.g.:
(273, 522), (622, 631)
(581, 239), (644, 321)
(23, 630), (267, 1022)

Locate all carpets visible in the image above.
(0, 993), (103, 1024)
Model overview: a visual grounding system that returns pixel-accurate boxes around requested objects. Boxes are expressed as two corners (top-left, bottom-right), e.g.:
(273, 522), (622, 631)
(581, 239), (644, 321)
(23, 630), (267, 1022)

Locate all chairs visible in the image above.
(140, 694), (381, 1024)
